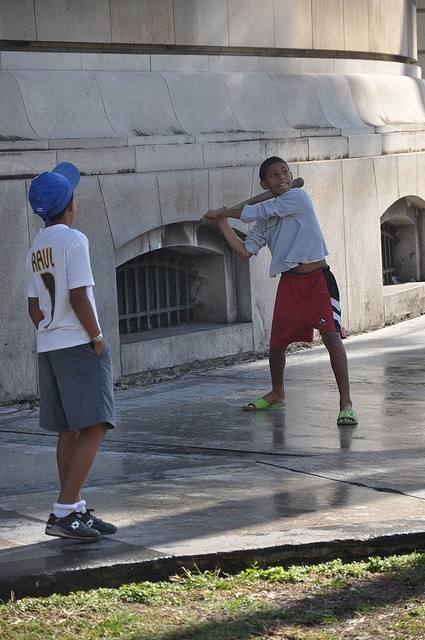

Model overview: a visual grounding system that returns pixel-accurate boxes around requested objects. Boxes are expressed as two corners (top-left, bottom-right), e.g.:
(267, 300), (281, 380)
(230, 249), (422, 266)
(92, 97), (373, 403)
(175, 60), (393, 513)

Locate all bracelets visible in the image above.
(91, 332), (104, 343)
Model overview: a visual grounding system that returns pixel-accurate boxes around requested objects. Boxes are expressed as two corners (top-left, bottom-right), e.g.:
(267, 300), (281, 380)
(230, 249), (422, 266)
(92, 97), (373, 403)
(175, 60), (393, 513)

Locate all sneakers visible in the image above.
(43, 511), (103, 543)
(76, 508), (117, 536)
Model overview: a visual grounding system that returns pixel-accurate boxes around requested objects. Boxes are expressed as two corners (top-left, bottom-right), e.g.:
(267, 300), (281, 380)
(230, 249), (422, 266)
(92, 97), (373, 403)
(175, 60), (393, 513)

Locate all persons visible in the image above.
(24, 162), (116, 544)
(205, 156), (359, 427)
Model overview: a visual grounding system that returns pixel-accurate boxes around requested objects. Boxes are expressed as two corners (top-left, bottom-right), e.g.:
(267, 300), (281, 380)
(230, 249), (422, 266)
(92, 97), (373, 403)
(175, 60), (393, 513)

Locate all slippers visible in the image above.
(241, 397), (286, 411)
(337, 408), (358, 425)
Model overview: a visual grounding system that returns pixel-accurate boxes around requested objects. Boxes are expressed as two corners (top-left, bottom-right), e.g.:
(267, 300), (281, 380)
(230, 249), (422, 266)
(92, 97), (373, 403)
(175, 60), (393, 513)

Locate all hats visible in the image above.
(28, 161), (81, 226)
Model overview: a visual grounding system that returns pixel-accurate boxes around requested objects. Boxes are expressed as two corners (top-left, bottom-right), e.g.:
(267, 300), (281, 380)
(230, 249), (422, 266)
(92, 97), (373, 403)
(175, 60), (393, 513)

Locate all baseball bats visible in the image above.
(200, 177), (305, 225)
